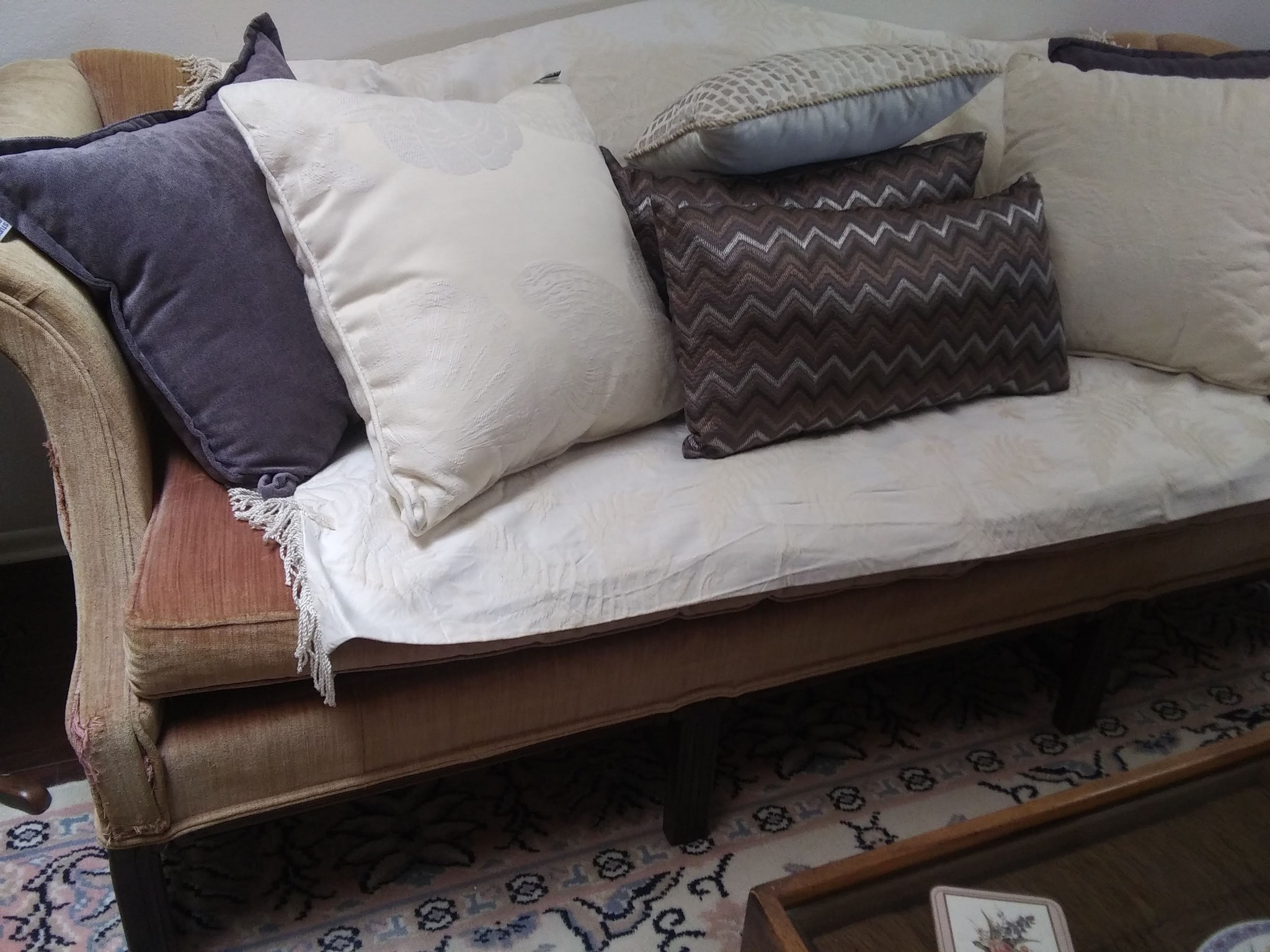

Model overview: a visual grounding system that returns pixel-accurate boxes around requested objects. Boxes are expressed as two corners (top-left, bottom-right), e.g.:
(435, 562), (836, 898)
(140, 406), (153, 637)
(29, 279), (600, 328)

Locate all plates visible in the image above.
(1195, 918), (1270, 952)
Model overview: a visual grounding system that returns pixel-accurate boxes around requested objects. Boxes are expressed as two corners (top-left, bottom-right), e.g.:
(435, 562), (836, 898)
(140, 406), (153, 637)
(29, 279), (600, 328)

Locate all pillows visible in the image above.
(598, 129), (985, 319)
(993, 52), (1268, 400)
(625, 42), (1004, 172)
(1047, 38), (1269, 78)
(213, 70), (679, 537)
(646, 172), (1069, 458)
(0, 13), (372, 499)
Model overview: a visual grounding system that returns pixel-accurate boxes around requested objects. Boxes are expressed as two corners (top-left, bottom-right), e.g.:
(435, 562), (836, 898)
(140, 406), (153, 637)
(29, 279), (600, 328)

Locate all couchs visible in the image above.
(0, 27), (1267, 952)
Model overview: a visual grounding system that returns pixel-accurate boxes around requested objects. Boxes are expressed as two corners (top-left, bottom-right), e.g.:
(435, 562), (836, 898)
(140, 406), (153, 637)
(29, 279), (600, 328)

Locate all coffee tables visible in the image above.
(740, 734), (1269, 952)
